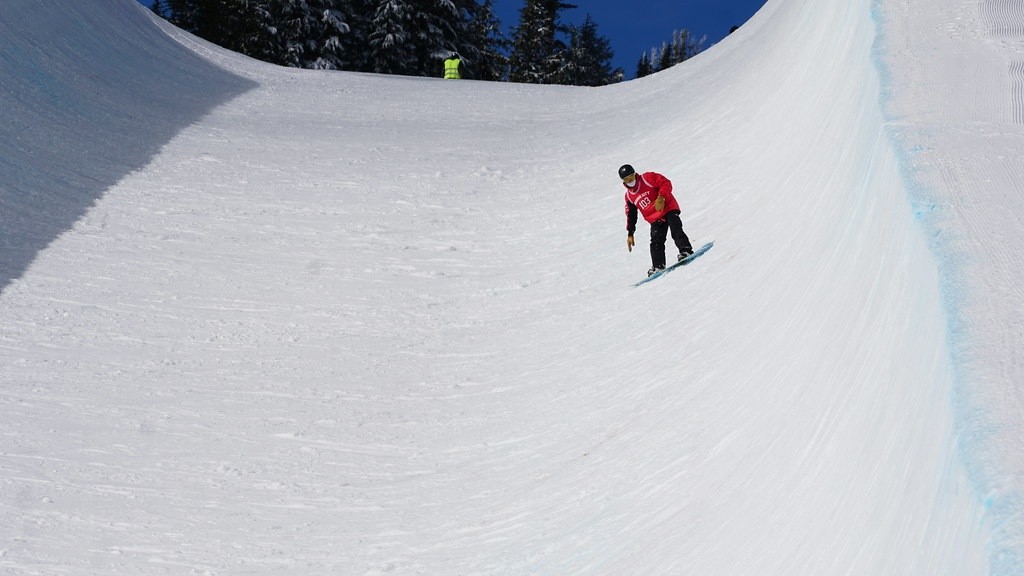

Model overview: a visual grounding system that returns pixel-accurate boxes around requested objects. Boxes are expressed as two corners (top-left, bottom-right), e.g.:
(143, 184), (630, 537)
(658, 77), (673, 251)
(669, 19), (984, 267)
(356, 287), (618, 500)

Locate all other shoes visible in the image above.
(677, 251), (693, 261)
(648, 265), (667, 277)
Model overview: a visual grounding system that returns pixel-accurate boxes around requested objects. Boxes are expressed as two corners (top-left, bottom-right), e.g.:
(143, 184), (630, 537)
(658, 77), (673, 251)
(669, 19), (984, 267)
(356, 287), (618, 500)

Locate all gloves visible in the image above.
(628, 236), (634, 252)
(655, 196), (664, 210)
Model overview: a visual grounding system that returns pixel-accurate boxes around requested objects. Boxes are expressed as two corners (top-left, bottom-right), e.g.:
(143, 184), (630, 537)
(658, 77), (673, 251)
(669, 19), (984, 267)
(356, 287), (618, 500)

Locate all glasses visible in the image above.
(624, 174), (635, 183)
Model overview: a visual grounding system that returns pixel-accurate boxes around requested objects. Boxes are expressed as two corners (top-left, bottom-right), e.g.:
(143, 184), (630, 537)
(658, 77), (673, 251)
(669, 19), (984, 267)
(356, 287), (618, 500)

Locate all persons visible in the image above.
(440, 52), (466, 80)
(618, 164), (694, 277)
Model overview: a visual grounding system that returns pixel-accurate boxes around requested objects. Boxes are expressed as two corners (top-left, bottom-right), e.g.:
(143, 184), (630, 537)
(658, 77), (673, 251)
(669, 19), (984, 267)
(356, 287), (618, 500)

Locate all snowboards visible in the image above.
(632, 241), (715, 286)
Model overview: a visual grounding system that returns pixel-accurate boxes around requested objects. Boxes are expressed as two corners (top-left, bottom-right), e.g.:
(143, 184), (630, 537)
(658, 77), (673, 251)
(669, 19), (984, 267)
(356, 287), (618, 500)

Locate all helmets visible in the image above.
(451, 54), (458, 57)
(619, 164), (635, 179)
